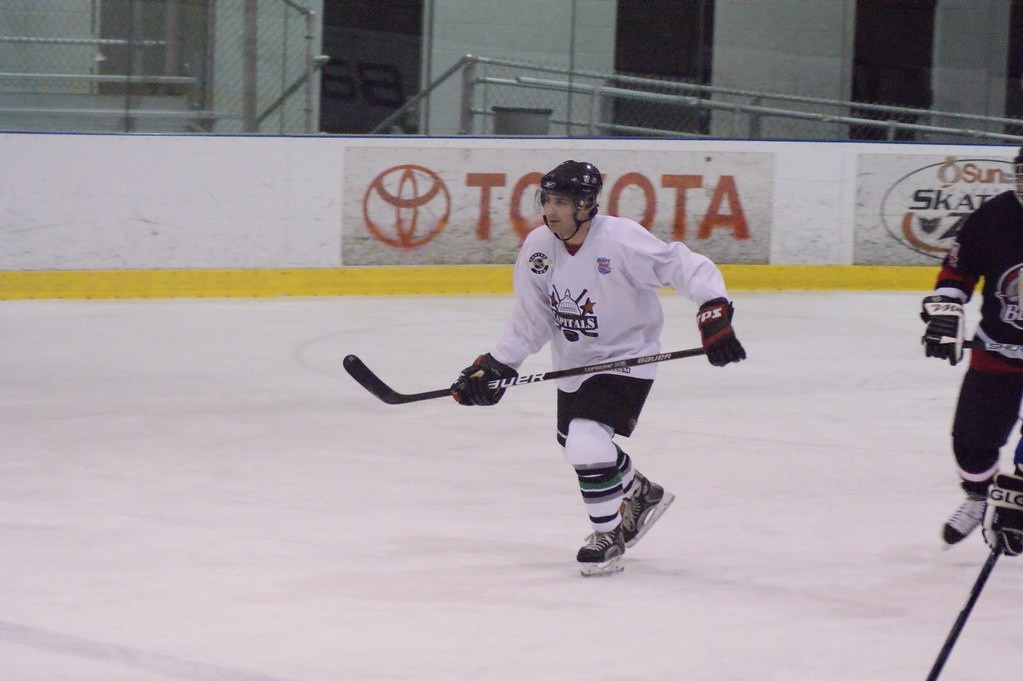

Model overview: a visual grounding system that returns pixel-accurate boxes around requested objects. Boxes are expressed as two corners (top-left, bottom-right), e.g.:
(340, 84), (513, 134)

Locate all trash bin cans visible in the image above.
(490, 104), (555, 136)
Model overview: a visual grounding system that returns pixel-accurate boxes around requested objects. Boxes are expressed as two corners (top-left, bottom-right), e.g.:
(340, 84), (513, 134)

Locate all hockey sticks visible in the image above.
(342, 346), (705, 405)
(921, 336), (1023, 353)
(926, 550), (1001, 681)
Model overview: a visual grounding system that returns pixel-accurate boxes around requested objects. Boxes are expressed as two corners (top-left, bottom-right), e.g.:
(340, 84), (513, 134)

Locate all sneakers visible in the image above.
(620, 469), (675, 548)
(942, 483), (988, 550)
(576, 522), (626, 576)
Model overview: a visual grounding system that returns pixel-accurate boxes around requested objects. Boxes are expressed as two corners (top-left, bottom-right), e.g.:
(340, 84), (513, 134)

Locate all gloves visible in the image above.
(450, 352), (519, 407)
(921, 295), (966, 365)
(982, 473), (1023, 555)
(697, 297), (746, 365)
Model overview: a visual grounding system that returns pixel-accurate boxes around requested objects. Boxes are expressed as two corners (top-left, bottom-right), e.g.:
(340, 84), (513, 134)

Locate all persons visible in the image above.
(449, 159), (746, 577)
(920, 146), (1023, 556)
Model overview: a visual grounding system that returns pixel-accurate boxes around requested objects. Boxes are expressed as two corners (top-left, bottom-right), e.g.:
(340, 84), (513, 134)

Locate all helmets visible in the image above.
(541, 159), (602, 208)
(1012, 147), (1023, 203)
